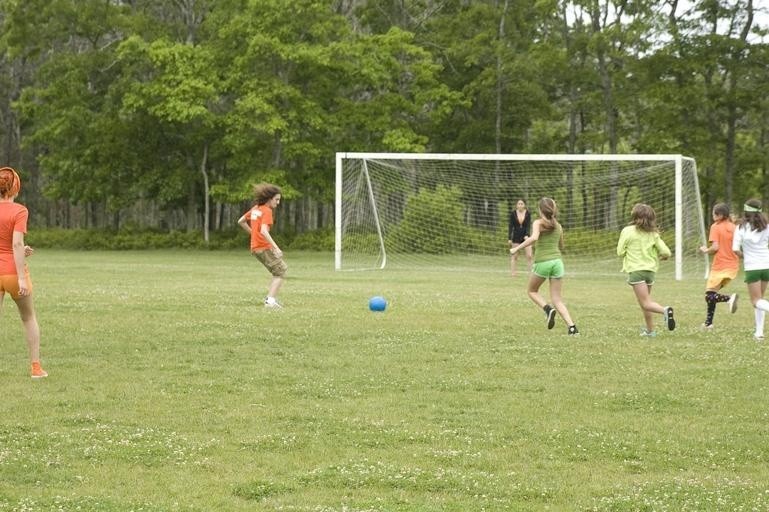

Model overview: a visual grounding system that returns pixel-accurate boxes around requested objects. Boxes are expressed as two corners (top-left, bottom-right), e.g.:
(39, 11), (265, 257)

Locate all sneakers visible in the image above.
(753, 334), (765, 342)
(30, 367), (48, 378)
(727, 293), (739, 313)
(664, 306), (676, 331)
(639, 329), (657, 338)
(263, 297), (285, 309)
(568, 325), (580, 337)
(546, 307), (557, 330)
(694, 323), (713, 334)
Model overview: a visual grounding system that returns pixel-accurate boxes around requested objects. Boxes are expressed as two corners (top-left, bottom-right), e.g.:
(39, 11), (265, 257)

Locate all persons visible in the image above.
(506, 199), (532, 274)
(0, 168), (47, 380)
(236, 183), (289, 306)
(732, 197), (769, 339)
(616, 201), (676, 340)
(699, 202), (739, 332)
(508, 196), (579, 336)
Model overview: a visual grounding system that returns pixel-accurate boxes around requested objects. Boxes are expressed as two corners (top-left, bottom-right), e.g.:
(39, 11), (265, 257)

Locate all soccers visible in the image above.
(369, 296), (387, 311)
